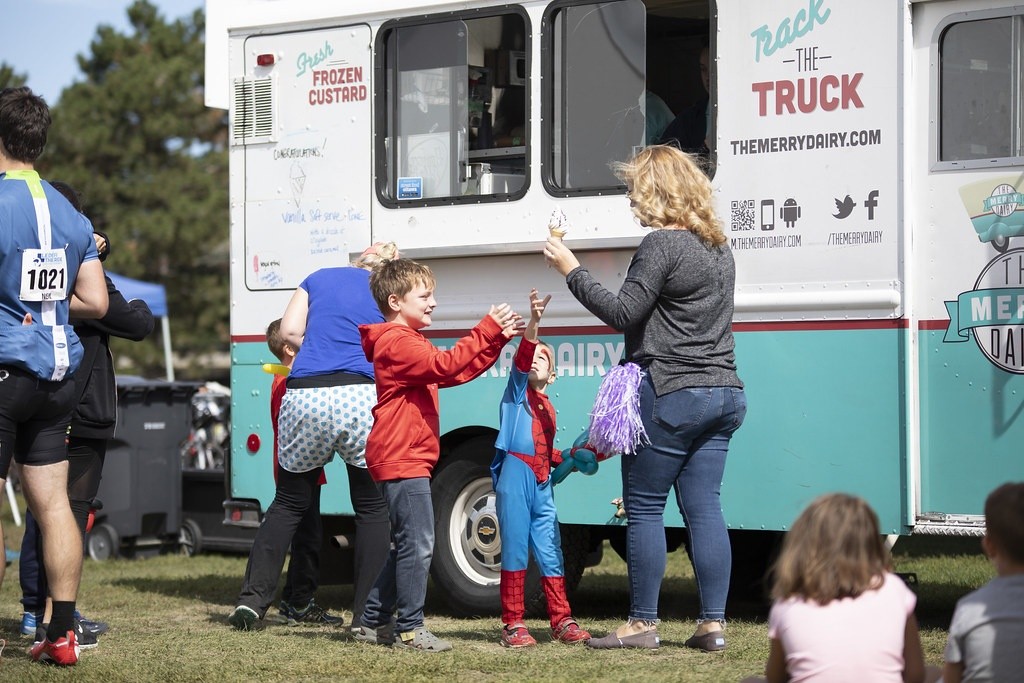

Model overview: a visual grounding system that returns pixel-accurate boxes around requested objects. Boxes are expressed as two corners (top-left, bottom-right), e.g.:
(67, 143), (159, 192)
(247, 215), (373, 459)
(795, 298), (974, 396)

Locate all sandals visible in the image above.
(356, 625), (399, 645)
(391, 626), (452, 654)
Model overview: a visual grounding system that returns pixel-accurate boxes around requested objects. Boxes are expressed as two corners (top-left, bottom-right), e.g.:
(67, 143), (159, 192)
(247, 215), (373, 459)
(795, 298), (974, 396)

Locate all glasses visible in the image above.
(624, 187), (633, 196)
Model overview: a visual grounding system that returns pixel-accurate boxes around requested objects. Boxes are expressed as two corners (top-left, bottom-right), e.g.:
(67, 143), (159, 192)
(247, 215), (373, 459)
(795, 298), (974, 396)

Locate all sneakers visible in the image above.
(34, 618), (99, 650)
(26, 631), (81, 665)
(500, 621), (537, 649)
(228, 605), (259, 631)
(20, 610), (108, 639)
(548, 616), (591, 644)
(278, 598), (343, 628)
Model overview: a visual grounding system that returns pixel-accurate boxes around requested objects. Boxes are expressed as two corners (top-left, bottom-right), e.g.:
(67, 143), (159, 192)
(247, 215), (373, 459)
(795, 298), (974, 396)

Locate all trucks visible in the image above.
(203, 1), (1023, 619)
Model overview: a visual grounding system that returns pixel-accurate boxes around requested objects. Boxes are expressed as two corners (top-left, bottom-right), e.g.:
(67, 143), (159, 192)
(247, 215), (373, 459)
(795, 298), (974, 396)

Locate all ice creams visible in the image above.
(548, 206), (569, 240)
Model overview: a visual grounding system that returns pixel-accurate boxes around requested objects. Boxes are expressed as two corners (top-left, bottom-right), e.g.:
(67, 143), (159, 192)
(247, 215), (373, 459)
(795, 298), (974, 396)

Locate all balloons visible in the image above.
(262, 364), (290, 377)
(552, 424), (599, 487)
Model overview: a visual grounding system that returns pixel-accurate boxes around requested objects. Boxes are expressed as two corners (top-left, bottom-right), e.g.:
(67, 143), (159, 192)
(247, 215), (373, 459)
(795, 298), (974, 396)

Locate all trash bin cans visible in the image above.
(86, 382), (201, 562)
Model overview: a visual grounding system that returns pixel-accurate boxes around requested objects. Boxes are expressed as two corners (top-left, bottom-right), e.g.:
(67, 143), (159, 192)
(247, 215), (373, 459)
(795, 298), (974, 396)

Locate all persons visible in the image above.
(543, 145), (748, 651)
(490, 287), (592, 648)
(227, 242), (525, 652)
(0, 85), (155, 666)
(741, 481), (1024, 683)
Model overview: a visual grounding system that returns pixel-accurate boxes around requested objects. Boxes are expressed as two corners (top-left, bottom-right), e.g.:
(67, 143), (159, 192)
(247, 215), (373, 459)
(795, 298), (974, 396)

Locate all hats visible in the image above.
(363, 242), (399, 264)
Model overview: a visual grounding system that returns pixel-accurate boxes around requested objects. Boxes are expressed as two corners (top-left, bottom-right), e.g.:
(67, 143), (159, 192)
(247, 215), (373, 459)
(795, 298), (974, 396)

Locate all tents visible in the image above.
(95, 270), (174, 384)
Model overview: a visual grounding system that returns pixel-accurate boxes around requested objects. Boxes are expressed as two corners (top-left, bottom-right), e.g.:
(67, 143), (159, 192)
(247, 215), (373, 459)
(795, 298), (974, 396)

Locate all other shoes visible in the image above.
(586, 629), (660, 651)
(685, 630), (726, 651)
